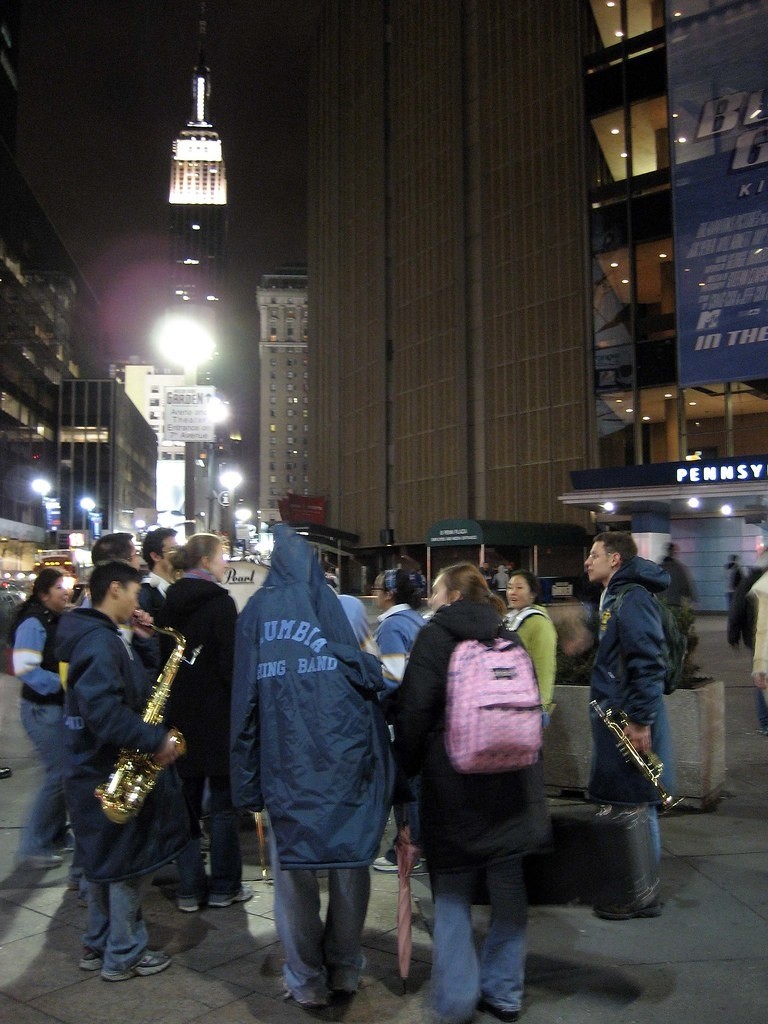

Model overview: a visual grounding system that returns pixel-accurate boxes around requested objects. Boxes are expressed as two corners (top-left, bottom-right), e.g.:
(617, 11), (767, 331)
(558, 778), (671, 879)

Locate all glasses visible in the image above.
(123, 550), (141, 560)
(369, 586), (387, 592)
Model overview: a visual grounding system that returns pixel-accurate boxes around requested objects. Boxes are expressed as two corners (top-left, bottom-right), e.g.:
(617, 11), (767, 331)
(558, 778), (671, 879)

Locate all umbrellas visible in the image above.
(393, 823), (421, 996)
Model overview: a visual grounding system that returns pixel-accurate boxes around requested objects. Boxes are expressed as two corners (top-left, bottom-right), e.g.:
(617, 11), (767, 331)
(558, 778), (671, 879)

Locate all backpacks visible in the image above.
(616, 582), (685, 695)
(444, 632), (541, 773)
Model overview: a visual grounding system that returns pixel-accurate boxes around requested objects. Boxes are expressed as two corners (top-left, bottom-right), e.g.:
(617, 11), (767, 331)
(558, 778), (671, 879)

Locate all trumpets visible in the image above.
(590, 697), (684, 816)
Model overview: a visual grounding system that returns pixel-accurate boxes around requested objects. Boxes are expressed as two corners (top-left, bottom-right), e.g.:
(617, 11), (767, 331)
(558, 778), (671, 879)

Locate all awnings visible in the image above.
(425, 518), (586, 566)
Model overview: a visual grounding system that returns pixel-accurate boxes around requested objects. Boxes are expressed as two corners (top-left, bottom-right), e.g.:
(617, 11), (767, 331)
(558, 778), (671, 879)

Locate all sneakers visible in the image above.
(208, 885), (252, 907)
(101, 948), (173, 980)
(80, 947), (104, 971)
(179, 895), (208, 911)
(593, 893), (661, 919)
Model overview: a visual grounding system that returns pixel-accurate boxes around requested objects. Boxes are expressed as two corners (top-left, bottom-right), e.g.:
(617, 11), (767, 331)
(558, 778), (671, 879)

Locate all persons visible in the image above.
(384, 562), (426, 610)
(230, 525), (395, 1010)
(323, 558), (380, 659)
(51, 527), (195, 980)
(358, 564), (375, 595)
(490, 562), (517, 599)
(357, 563), (430, 874)
(380, 559), (553, 1024)
(497, 571), (559, 847)
(480, 561), (494, 589)
(584, 529), (671, 919)
(651, 543), (700, 677)
(154, 535), (254, 913)
(6, 568), (74, 869)
(722, 532), (768, 732)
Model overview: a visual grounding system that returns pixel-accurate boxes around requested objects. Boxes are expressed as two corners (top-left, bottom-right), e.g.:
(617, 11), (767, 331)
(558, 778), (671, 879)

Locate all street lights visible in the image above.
(219, 462), (241, 559)
(78, 493), (96, 551)
(29, 476), (53, 550)
(145, 297), (218, 540)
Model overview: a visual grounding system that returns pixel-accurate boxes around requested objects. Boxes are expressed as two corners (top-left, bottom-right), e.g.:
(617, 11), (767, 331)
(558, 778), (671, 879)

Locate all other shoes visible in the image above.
(14, 852), (62, 867)
(482, 999), (518, 1021)
(374, 856), (422, 870)
(53, 829), (76, 850)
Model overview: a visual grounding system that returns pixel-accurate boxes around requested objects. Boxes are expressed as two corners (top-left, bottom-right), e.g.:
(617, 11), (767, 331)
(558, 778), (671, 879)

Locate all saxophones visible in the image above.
(95, 610), (207, 824)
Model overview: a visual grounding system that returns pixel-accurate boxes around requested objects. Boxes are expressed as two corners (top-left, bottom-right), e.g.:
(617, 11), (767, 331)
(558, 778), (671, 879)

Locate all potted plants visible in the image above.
(539, 595), (728, 811)
